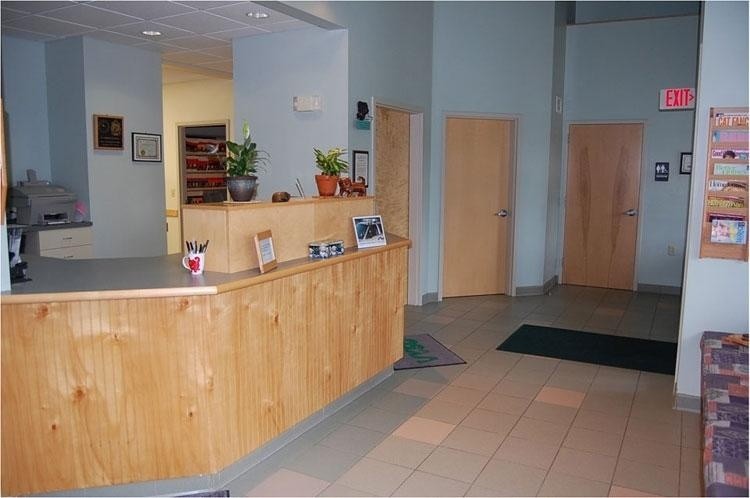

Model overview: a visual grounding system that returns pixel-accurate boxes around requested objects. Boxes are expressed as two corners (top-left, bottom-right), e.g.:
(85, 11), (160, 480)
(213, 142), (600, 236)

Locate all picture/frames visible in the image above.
(678, 152), (691, 174)
(352, 215), (388, 250)
(92, 112), (126, 152)
(252, 229), (279, 277)
(131, 131), (164, 164)
(351, 150), (370, 189)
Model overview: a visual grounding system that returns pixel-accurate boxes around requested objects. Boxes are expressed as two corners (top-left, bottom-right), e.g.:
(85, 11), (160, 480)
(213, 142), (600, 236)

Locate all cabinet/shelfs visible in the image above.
(185, 135), (228, 197)
(22, 227), (92, 262)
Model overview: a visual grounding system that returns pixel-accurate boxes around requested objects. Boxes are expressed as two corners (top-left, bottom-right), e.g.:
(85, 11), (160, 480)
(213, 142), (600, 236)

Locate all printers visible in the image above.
(7, 169), (77, 226)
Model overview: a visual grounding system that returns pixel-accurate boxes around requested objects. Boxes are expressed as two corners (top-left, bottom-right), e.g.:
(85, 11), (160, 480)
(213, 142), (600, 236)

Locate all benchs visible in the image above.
(699, 330), (749, 497)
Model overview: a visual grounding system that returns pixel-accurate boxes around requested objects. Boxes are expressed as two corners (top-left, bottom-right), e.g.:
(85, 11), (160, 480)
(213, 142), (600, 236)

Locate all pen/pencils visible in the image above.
(186, 239), (210, 254)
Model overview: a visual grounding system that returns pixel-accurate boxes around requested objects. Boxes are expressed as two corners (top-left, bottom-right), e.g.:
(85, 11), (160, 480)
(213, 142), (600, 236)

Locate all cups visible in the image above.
(181, 253), (204, 276)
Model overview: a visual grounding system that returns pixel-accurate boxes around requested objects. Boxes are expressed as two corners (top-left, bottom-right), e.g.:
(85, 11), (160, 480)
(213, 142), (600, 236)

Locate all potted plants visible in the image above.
(313, 144), (352, 196)
(221, 120), (271, 204)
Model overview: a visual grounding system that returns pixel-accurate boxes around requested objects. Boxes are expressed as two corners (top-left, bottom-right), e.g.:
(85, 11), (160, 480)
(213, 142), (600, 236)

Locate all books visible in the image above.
(708, 110), (749, 245)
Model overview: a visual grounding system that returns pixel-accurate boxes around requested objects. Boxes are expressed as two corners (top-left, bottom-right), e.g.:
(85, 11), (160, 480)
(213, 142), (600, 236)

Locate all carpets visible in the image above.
(494, 320), (678, 377)
(390, 331), (466, 374)
